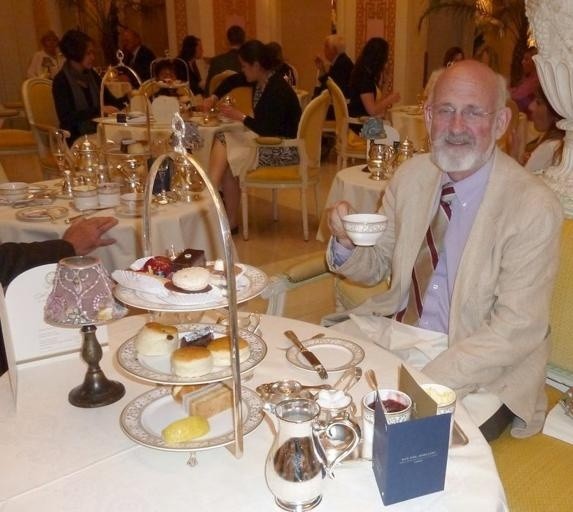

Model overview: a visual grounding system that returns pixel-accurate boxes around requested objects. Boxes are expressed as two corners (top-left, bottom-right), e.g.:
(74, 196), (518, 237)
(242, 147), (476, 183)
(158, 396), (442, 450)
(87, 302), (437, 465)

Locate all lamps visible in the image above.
(44, 255), (127, 407)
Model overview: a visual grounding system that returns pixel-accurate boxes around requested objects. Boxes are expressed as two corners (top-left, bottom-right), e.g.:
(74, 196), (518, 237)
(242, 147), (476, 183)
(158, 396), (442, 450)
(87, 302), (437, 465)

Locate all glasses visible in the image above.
(429, 103), (498, 122)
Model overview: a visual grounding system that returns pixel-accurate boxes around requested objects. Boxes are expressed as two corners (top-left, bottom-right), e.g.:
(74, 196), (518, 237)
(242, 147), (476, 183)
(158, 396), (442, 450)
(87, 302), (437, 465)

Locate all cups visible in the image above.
(418, 383), (457, 449)
(406, 106), (418, 115)
(116, 113), (126, 123)
(0, 131), (204, 215)
(361, 390), (411, 459)
(340, 212), (390, 246)
(311, 391), (358, 422)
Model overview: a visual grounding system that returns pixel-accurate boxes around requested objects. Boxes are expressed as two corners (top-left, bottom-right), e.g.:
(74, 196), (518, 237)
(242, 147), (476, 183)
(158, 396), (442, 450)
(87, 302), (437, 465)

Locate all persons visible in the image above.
(0, 216), (119, 375)
(504, 50), (565, 174)
(26, 30), (68, 77)
(420, 46), (465, 99)
(51, 24), (301, 237)
(315, 34), (403, 160)
(325, 61), (566, 441)
(476, 46), (500, 72)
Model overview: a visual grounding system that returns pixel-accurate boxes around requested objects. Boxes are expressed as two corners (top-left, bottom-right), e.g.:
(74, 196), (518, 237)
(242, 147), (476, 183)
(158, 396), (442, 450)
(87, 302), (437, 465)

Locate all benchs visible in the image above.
(268, 198), (570, 509)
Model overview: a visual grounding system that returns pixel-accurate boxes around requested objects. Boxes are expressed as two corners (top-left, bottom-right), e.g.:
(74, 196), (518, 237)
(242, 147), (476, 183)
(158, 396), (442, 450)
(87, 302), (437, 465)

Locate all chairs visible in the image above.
(5, 264), (107, 358)
(0, 64), (528, 262)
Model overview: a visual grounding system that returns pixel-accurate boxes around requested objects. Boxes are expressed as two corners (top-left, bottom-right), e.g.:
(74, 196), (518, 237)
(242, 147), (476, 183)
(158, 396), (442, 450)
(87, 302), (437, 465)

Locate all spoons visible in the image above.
(271, 379), (333, 393)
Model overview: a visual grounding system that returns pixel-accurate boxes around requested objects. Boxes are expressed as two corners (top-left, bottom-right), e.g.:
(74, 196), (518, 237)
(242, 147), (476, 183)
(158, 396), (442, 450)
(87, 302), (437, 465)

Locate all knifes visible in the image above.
(286, 331), (328, 380)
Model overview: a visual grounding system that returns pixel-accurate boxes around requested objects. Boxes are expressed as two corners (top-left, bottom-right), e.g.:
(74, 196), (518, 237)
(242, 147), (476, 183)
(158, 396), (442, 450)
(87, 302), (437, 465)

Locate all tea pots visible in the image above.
(221, 309), (263, 385)
(198, 106), (217, 126)
(261, 399), (359, 511)
(365, 144), (395, 183)
(393, 137), (424, 173)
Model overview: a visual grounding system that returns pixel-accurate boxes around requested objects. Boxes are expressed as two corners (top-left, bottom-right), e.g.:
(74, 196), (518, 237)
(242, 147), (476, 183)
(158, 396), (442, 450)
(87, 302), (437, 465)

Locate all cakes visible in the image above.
(132, 318), (251, 378)
(114, 246), (243, 305)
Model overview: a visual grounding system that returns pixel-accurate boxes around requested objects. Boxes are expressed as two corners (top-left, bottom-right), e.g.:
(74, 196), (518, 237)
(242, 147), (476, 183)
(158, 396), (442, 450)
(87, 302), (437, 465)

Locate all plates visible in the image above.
(286, 336), (364, 370)
(16, 204), (67, 221)
(120, 384), (264, 449)
(117, 322), (269, 384)
(110, 262), (269, 313)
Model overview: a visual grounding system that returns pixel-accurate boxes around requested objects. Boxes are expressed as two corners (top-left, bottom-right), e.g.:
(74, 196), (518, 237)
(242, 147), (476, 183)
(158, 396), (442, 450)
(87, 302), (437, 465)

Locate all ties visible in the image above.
(386, 183), (456, 326)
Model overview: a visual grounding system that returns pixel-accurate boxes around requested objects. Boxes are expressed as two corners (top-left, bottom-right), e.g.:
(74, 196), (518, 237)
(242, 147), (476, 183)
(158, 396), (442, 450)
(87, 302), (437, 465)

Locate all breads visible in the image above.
(171, 385), (234, 420)
(162, 415), (209, 444)
